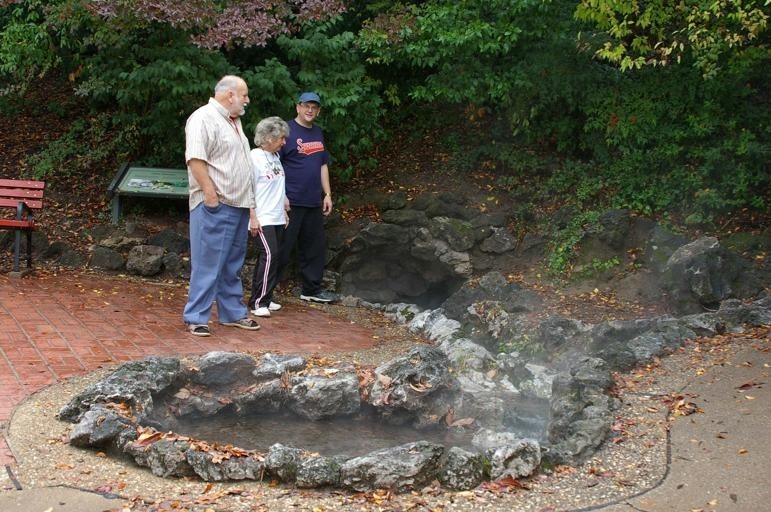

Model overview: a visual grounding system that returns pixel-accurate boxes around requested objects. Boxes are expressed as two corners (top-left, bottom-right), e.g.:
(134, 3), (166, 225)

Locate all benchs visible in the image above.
(0, 179), (45, 279)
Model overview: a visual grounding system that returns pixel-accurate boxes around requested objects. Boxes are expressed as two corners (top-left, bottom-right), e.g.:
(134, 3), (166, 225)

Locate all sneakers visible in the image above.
(250, 306), (271, 317)
(268, 301), (282, 311)
(300, 292), (333, 302)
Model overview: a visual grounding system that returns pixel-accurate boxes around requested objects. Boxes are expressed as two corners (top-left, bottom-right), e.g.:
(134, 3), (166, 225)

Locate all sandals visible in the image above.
(222, 317), (262, 331)
(185, 322), (211, 336)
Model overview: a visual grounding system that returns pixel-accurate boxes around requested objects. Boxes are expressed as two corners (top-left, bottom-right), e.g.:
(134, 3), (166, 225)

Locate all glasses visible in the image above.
(299, 103), (319, 112)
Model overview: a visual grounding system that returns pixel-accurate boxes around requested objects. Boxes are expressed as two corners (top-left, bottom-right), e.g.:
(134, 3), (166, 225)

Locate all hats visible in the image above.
(299, 92), (321, 105)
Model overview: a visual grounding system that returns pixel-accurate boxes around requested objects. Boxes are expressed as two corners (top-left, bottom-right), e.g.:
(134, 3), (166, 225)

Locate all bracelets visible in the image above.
(325, 192), (332, 196)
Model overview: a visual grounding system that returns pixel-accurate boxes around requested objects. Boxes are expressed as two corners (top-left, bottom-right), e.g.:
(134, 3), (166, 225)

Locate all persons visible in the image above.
(275, 92), (335, 303)
(185, 75), (261, 336)
(248, 117), (289, 316)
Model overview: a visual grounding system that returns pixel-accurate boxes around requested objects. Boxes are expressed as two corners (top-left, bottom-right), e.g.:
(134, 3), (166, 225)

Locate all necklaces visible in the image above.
(261, 145), (276, 165)
